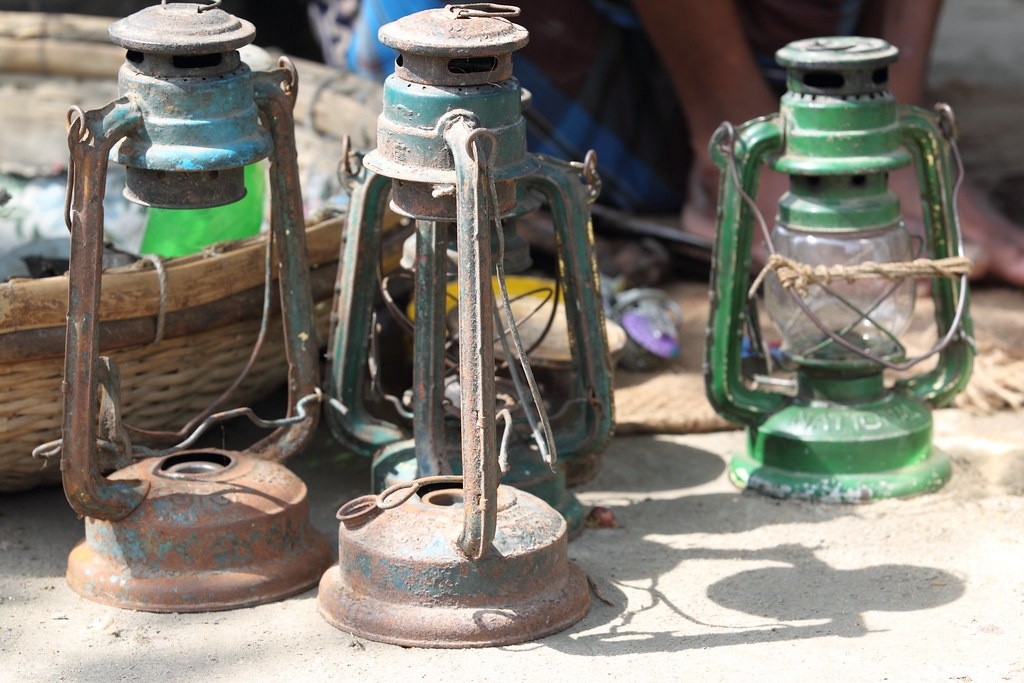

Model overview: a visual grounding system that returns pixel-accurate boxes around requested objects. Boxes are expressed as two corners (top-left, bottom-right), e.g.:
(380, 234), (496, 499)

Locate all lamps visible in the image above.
(705, 34), (980, 504)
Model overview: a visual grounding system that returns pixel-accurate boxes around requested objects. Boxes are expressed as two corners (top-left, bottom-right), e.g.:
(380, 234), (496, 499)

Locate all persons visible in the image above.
(342, 0), (1024, 289)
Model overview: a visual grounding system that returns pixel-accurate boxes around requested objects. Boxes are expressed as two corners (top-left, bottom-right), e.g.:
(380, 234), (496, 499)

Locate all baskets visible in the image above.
(0, 12), (415, 498)
